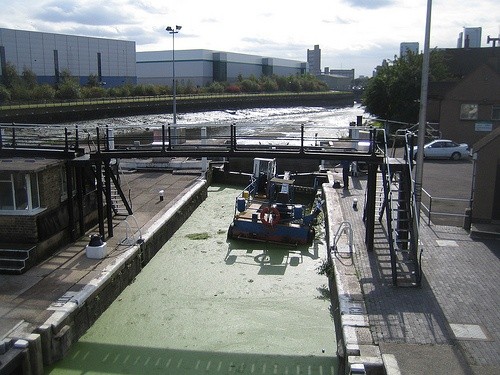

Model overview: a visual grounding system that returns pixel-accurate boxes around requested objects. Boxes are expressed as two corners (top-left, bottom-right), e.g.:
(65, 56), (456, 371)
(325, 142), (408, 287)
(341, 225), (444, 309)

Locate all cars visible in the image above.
(407, 139), (470, 162)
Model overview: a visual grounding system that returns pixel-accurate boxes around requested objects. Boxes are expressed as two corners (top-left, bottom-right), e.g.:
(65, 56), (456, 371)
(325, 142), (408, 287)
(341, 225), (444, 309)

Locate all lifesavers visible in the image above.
(260, 207), (280, 226)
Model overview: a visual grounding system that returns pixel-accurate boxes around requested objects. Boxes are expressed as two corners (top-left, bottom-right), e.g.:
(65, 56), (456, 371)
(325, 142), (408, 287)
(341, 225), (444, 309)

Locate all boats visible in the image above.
(227, 158), (318, 247)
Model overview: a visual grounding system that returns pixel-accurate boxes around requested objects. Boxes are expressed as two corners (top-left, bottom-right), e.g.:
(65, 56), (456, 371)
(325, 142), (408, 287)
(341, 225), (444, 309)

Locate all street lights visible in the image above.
(166, 26), (182, 144)
